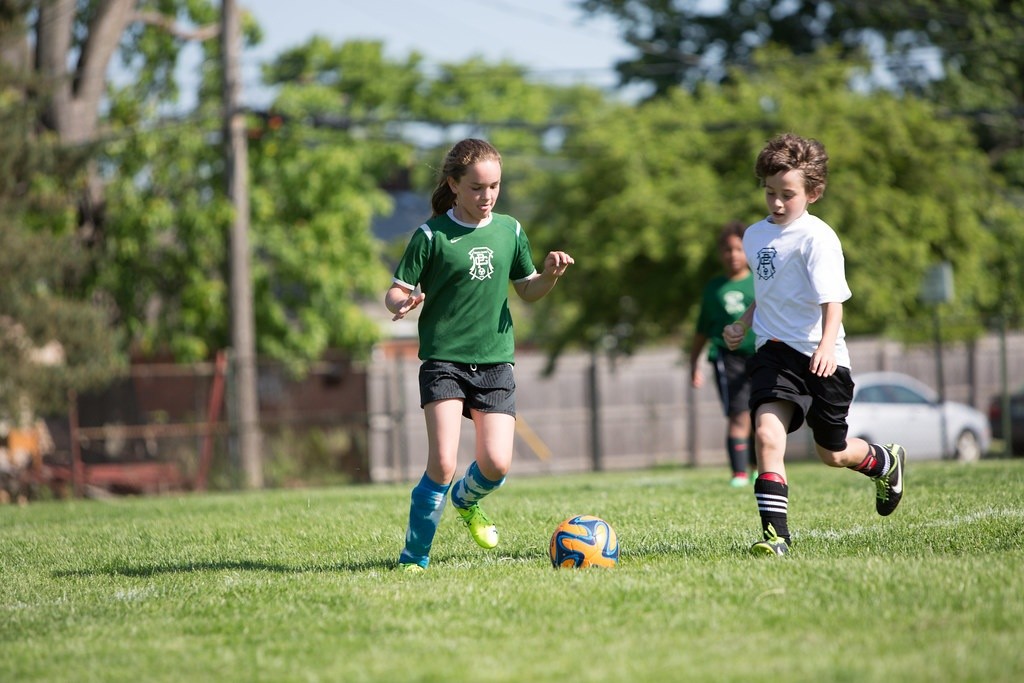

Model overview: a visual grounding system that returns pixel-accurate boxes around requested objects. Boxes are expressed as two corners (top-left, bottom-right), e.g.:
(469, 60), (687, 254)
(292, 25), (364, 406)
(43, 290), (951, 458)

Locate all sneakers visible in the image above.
(452, 501), (498, 549)
(398, 562), (423, 573)
(751, 523), (790, 557)
(870, 444), (905, 516)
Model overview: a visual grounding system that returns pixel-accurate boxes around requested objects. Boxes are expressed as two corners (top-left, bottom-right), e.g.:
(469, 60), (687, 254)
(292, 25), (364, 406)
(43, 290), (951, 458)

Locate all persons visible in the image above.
(0, 396), (56, 504)
(691, 222), (757, 487)
(387, 138), (575, 576)
(744, 133), (908, 557)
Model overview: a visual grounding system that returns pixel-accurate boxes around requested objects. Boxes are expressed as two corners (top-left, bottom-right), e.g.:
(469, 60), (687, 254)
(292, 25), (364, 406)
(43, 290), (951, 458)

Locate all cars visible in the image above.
(988, 389), (1024, 460)
(784, 368), (993, 462)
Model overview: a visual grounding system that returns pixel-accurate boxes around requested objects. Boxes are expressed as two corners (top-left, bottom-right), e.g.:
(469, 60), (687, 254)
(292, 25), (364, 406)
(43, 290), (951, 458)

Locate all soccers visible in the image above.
(548, 513), (621, 572)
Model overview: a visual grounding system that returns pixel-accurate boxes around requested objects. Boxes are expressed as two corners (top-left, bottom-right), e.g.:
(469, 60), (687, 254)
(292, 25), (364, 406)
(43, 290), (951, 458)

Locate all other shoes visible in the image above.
(751, 473), (758, 486)
(732, 475), (748, 487)
